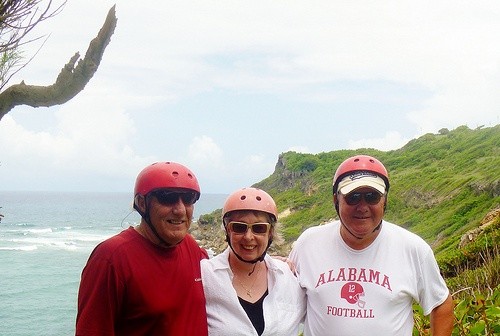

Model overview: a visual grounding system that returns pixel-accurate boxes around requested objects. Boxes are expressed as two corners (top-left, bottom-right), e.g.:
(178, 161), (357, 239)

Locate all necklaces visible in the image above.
(227, 260), (260, 298)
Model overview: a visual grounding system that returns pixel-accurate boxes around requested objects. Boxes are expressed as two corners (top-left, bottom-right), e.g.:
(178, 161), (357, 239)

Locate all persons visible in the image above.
(75, 161), (210, 336)
(199, 187), (309, 336)
(288, 155), (454, 336)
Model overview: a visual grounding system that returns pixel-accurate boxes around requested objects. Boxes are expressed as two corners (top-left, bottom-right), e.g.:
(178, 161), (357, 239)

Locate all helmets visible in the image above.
(222, 188), (278, 231)
(333, 155), (389, 192)
(133, 162), (200, 208)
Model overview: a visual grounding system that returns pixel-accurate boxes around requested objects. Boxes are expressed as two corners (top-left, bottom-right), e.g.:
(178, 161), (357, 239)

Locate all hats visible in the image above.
(336, 171), (386, 196)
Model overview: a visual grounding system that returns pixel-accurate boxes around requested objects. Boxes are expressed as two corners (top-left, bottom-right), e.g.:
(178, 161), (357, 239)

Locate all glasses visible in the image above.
(151, 190), (197, 205)
(344, 192), (383, 205)
(225, 221), (271, 234)
